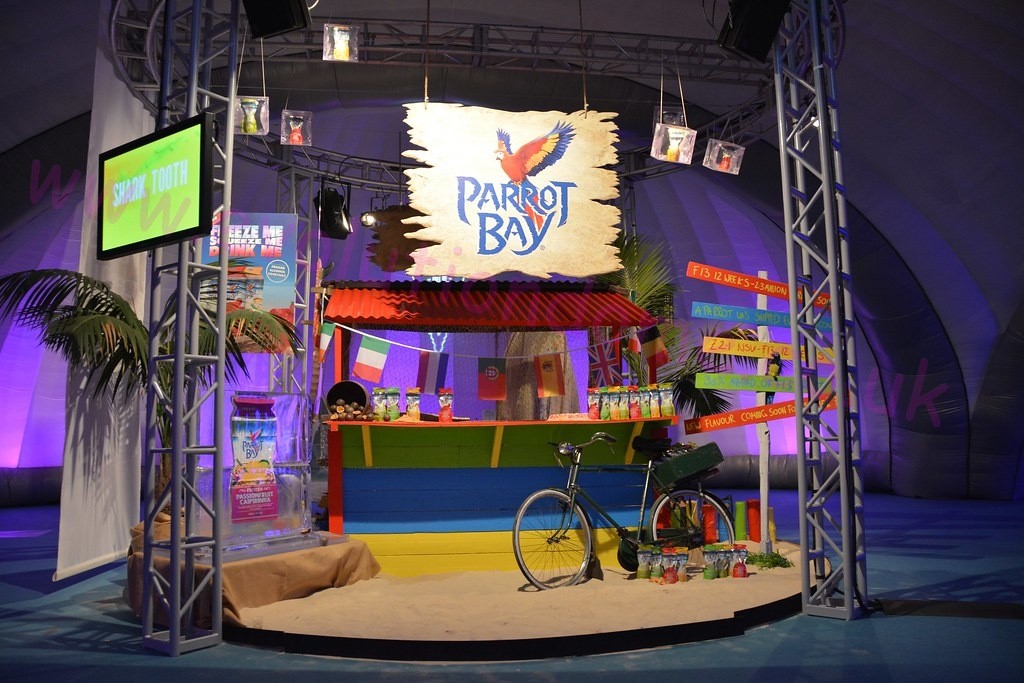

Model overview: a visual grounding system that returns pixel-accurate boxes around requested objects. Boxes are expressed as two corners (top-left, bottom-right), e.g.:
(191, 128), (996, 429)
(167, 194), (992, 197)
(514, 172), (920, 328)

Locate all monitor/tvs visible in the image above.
(96, 111), (214, 260)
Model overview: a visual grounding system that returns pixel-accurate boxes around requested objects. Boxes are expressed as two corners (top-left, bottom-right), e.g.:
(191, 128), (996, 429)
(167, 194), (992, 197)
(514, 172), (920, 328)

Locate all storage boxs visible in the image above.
(652, 442), (724, 487)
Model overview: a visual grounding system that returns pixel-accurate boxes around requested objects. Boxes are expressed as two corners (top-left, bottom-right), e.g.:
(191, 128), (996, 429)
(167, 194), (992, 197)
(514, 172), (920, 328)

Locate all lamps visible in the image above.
(313, 174), (354, 241)
(360, 193), (387, 228)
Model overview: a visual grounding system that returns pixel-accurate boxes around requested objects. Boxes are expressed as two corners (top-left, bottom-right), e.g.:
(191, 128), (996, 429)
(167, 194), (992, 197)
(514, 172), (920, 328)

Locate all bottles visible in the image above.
(437, 388), (454, 424)
(721, 152), (731, 171)
(230, 398), (278, 488)
(667, 133), (684, 162)
(702, 544), (749, 579)
(637, 545), (688, 583)
(333, 26), (351, 61)
(406, 387), (421, 422)
(240, 99), (259, 134)
(385, 388), (401, 422)
(372, 386), (386, 420)
(287, 116), (305, 144)
(587, 383), (674, 418)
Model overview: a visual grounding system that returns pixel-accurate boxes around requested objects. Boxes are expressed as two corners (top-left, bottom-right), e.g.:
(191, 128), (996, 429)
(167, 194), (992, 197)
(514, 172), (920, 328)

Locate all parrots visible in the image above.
(764, 352), (782, 405)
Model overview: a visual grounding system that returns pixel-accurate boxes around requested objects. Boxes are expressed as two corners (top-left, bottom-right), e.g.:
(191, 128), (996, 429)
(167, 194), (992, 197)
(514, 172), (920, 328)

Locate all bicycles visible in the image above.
(511, 432), (736, 591)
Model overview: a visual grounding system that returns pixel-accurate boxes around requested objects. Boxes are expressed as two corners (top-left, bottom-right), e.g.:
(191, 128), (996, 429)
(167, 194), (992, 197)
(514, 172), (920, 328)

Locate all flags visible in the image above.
(352, 336), (390, 384)
(636, 327), (671, 370)
(534, 354), (565, 399)
(319, 323), (337, 360)
(589, 341), (624, 388)
(416, 351), (449, 397)
(478, 357), (508, 401)
